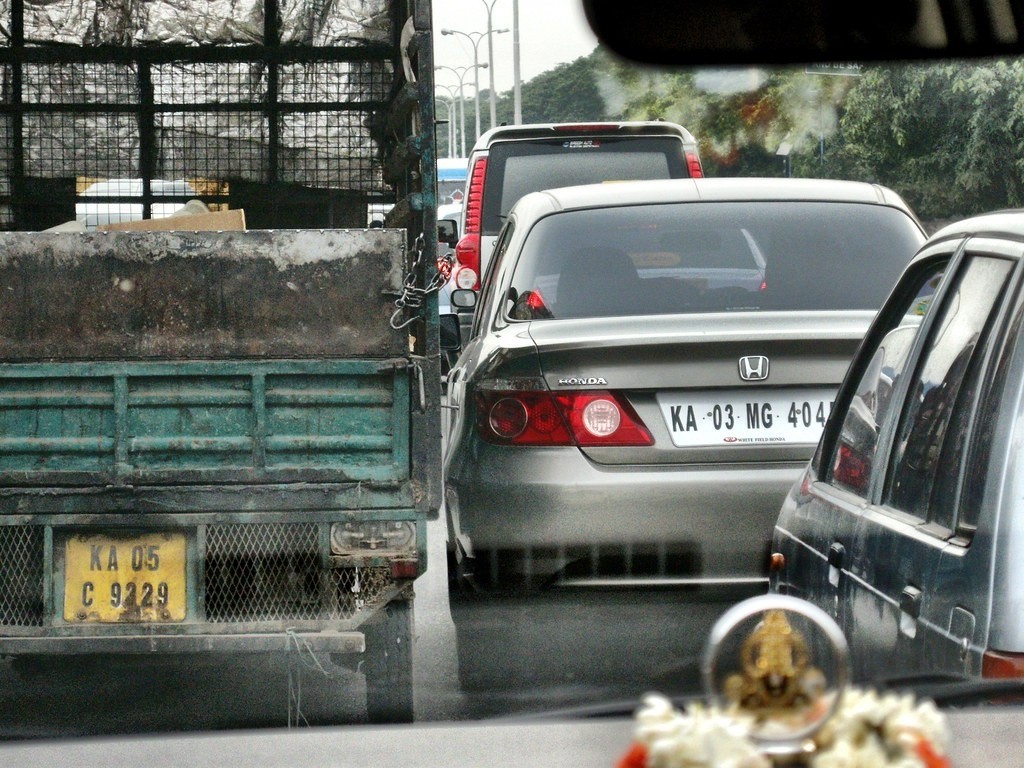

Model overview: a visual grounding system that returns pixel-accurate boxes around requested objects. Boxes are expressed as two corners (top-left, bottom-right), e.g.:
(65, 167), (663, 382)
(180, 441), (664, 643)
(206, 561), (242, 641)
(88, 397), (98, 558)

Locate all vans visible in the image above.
(437, 121), (707, 350)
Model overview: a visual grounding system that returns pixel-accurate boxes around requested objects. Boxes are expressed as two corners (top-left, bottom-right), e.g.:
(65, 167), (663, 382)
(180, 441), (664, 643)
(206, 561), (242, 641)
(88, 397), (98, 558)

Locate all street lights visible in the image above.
(434, 63), (488, 158)
(435, 96), (472, 158)
(442, 28), (510, 142)
(436, 83), (475, 158)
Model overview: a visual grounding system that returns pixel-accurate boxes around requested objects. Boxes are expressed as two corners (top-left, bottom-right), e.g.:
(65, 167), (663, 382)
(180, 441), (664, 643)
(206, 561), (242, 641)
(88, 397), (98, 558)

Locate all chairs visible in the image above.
(763, 245), (845, 310)
(555, 247), (639, 318)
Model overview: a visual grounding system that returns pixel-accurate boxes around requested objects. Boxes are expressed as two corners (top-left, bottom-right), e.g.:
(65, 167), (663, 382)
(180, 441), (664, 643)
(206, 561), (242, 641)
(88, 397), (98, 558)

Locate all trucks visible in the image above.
(1, 1), (442, 724)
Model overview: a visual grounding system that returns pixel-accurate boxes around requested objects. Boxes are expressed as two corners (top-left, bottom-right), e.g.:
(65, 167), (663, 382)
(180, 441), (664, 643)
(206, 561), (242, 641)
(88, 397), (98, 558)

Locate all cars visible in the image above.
(769, 208), (1023, 689)
(440, 177), (973, 693)
